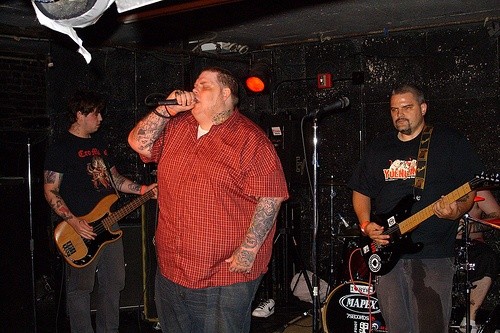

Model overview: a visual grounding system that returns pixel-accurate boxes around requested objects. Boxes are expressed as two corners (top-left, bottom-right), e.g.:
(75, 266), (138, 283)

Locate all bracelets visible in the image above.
(164, 106), (177, 116)
(152, 105), (172, 119)
(360, 221), (370, 236)
(140, 185), (146, 195)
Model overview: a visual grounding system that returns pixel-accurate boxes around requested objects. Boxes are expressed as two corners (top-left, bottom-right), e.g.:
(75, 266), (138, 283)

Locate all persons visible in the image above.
(128, 66), (289, 333)
(456, 186), (500, 333)
(347, 80), (483, 333)
(44, 97), (157, 333)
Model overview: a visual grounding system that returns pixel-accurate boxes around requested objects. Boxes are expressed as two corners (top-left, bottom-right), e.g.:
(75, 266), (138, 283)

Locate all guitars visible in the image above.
(360, 172), (500, 276)
(55, 185), (158, 268)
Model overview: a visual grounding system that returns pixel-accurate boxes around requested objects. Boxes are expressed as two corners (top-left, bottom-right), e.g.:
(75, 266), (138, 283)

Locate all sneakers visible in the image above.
(252, 298), (276, 319)
(460, 316), (482, 333)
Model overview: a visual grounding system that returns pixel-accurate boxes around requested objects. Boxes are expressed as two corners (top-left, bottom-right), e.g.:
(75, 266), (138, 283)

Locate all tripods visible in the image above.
(274, 114), (317, 316)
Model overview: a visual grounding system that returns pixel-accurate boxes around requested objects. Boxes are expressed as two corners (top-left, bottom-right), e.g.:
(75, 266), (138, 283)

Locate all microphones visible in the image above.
(148, 99), (179, 106)
(308, 96), (350, 118)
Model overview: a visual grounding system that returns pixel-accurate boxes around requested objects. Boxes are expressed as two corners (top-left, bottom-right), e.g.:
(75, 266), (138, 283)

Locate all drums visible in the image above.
(333, 227), (375, 280)
(455, 239), (489, 282)
(322, 278), (388, 333)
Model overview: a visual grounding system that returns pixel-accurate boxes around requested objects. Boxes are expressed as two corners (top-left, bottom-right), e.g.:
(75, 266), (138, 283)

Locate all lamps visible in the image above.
(244, 58), (270, 92)
